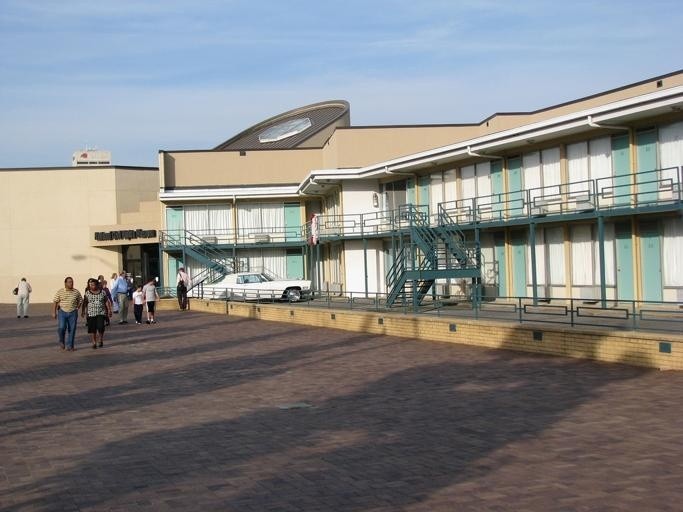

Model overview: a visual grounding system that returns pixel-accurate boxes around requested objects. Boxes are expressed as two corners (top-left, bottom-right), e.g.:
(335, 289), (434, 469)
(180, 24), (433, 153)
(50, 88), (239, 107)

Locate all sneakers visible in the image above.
(113, 311), (119, 313)
(17, 316), (20, 318)
(24, 316), (29, 318)
(92, 344), (96, 348)
(98, 341), (102, 346)
(57, 343), (64, 349)
(184, 309), (186, 311)
(118, 320), (156, 324)
(180, 309), (183, 311)
(66, 348), (74, 352)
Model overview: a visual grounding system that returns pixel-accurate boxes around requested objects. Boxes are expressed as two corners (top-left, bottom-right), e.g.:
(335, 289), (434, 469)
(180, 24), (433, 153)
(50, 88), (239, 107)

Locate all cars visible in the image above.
(192, 269), (313, 303)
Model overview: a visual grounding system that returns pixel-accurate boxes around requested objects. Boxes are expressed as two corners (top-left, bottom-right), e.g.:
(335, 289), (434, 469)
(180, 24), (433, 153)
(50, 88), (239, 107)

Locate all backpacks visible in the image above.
(177, 280), (185, 292)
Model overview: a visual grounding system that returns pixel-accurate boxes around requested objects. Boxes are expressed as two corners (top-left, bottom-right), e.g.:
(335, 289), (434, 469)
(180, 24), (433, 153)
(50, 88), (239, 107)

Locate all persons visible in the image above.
(309, 208), (317, 221)
(11, 267), (190, 353)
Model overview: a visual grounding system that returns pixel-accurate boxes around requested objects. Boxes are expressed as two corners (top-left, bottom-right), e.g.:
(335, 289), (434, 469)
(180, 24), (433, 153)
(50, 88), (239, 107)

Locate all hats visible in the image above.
(125, 272), (133, 277)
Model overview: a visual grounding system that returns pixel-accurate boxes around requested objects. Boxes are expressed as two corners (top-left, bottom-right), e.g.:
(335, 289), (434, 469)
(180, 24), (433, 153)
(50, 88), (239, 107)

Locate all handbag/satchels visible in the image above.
(13, 288), (18, 295)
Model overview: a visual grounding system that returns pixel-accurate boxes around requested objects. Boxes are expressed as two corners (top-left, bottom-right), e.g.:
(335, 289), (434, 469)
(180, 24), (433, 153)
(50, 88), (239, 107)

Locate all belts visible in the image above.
(59, 309), (76, 313)
(117, 292), (126, 294)
